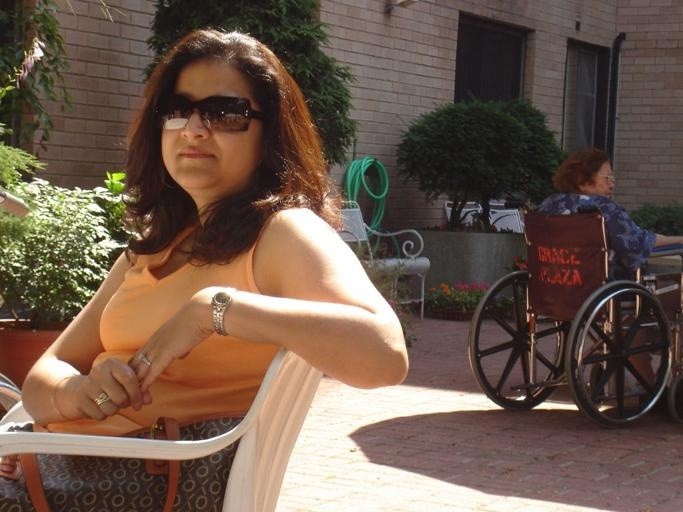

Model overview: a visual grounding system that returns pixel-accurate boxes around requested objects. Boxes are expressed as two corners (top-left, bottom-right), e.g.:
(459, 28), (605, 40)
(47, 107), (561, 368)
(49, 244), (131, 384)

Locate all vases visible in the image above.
(425, 308), (503, 321)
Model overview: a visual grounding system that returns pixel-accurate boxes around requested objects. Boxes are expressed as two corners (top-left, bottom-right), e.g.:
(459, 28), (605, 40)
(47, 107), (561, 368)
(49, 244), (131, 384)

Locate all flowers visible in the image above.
(428, 280), (499, 310)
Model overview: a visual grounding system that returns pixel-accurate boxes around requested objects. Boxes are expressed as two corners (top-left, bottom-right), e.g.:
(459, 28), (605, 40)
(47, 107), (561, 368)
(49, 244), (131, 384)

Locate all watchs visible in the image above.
(210, 284), (237, 337)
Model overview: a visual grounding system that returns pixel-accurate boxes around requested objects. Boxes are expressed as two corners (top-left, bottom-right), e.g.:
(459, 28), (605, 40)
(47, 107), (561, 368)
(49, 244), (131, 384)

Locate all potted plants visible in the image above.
(395, 97), (565, 297)
(0, 178), (111, 416)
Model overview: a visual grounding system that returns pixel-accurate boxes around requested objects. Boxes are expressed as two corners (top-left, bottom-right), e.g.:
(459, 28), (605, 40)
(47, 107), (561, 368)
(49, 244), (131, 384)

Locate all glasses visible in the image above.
(156, 93), (268, 132)
(596, 174), (615, 183)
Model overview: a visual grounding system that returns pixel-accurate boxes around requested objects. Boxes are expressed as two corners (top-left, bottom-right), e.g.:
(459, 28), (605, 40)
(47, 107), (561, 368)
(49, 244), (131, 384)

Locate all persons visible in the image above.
(540, 148), (683, 390)
(0, 30), (409, 442)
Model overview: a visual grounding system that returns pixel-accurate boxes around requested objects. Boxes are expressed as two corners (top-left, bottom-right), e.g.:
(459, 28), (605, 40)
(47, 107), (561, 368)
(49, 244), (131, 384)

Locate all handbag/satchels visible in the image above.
(2, 417), (246, 512)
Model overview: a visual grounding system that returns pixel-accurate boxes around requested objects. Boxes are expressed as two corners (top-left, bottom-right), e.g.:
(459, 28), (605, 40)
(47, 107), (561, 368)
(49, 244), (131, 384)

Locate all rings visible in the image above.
(138, 353), (153, 366)
(95, 391), (111, 406)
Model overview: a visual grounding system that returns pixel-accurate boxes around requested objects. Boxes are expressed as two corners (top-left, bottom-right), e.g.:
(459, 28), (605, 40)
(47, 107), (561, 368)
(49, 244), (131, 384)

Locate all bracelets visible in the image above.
(48, 375), (71, 419)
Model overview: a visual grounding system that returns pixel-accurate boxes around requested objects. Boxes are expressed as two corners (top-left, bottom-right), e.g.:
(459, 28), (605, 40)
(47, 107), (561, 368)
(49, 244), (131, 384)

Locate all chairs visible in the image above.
(0, 347), (325, 512)
(336, 201), (431, 321)
(445, 202), (481, 226)
(481, 203), (525, 233)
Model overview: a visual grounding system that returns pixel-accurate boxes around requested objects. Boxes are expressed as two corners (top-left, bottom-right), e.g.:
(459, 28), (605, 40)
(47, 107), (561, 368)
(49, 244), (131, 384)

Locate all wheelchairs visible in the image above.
(466, 208), (683, 431)
